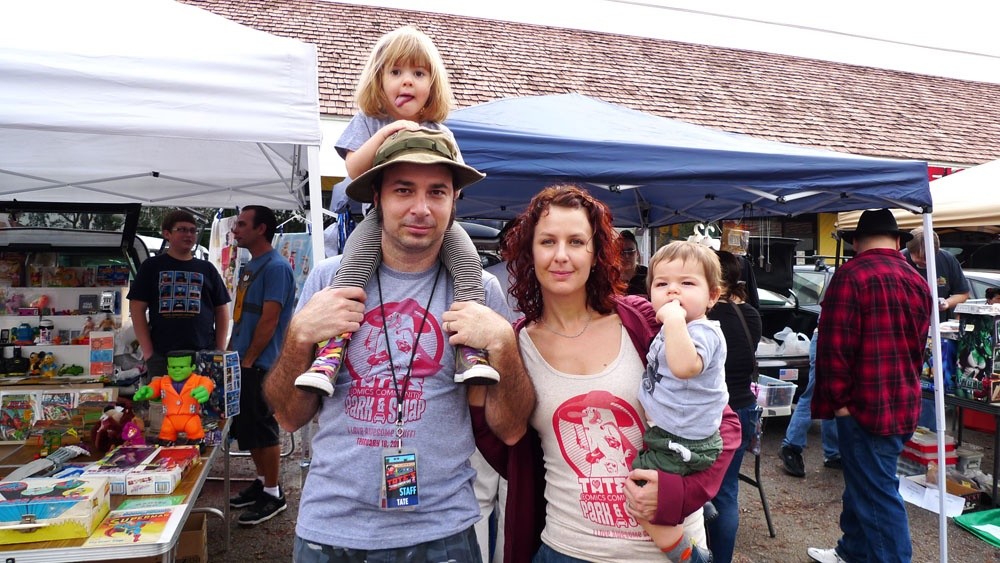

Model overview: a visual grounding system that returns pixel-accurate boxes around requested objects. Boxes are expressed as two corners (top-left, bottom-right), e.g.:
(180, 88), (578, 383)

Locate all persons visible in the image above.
(99, 314), (115, 331)
(264, 124), (535, 563)
(230, 205), (297, 527)
(80, 317), (94, 336)
(133, 350), (215, 454)
(293, 27), (500, 398)
(807, 209), (933, 563)
(985, 288), (1000, 305)
(127, 211), (231, 384)
(899, 226), (969, 327)
(777, 314), (845, 478)
(469, 181), (762, 563)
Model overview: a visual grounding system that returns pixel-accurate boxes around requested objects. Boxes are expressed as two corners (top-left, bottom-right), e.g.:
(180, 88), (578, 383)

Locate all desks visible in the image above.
(0, 417), (234, 563)
(921, 388), (1000, 503)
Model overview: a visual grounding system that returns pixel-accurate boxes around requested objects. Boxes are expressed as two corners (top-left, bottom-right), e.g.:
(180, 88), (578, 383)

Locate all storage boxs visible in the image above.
(195, 349), (240, 418)
(757, 374), (799, 418)
(920, 303), (1000, 403)
(0, 445), (201, 543)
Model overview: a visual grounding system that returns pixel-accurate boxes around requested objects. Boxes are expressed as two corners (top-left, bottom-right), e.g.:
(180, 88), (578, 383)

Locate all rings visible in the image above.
(628, 502), (630, 507)
(447, 322), (450, 332)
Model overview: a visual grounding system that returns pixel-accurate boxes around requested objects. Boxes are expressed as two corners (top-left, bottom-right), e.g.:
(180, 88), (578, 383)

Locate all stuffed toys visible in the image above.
(95, 405), (136, 452)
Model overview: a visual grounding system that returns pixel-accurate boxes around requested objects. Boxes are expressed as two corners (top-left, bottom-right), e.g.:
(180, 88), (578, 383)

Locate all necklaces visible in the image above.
(543, 312), (592, 339)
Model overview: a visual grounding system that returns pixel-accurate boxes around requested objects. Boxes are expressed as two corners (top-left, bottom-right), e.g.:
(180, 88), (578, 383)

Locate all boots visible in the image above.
(294, 332), (352, 398)
(455, 344), (501, 385)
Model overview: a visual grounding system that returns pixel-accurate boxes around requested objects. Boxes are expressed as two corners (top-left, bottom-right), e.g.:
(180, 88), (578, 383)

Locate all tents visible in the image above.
(0, 1), (325, 270)
(332, 94), (949, 563)
(836, 158), (1000, 270)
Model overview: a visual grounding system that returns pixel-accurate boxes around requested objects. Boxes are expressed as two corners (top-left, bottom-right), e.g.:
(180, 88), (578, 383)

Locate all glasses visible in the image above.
(171, 227), (200, 234)
(621, 249), (638, 256)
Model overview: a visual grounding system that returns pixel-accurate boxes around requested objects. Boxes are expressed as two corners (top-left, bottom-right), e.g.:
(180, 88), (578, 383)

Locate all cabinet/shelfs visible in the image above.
(0, 281), (132, 394)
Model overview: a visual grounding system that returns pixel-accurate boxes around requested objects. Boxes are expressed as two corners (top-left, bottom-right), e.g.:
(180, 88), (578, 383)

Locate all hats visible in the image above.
(837, 209), (914, 242)
(343, 125), (486, 202)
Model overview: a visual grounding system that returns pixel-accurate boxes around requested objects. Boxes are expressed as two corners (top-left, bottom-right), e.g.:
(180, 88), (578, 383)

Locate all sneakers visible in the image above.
(777, 444), (806, 477)
(237, 484), (287, 525)
(825, 458), (841, 468)
(806, 546), (847, 563)
(229, 479), (263, 507)
